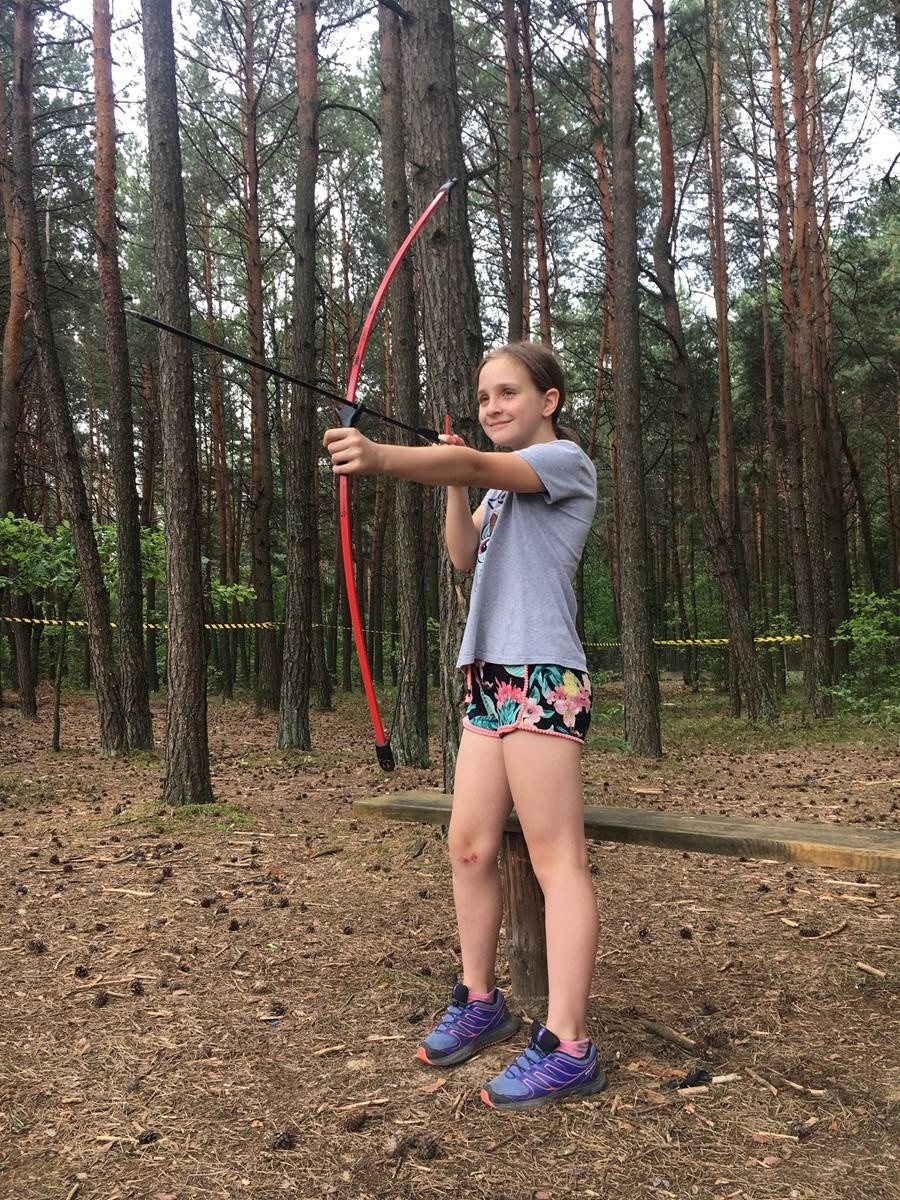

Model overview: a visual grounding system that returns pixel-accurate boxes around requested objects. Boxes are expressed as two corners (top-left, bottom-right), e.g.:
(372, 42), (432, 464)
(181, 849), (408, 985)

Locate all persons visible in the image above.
(321, 342), (606, 1112)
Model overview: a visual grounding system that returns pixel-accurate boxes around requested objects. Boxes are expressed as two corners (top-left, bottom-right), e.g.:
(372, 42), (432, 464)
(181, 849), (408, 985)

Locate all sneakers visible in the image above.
(480, 1019), (608, 1111)
(413, 983), (521, 1067)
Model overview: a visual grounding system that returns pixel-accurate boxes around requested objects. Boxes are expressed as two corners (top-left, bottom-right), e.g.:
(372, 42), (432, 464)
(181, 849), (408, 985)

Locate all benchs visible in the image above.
(355, 790), (900, 1002)
